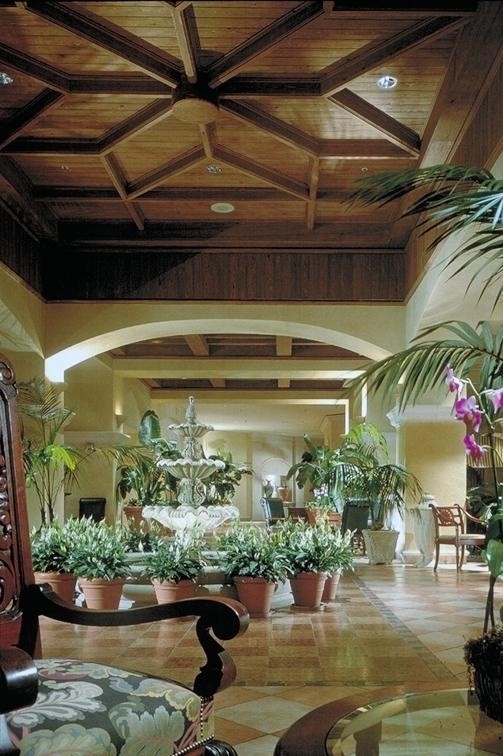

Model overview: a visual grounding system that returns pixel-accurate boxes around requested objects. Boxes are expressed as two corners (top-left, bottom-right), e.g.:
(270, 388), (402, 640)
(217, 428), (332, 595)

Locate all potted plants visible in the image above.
(28, 517), (75, 607)
(285, 431), (339, 528)
(104, 407), (181, 538)
(140, 524), (208, 623)
(73, 513), (135, 613)
(216, 524), (278, 618)
(336, 459), (425, 569)
(279, 520), (357, 614)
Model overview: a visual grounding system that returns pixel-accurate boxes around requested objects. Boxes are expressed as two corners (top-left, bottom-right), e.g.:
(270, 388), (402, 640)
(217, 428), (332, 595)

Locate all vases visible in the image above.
(463, 629), (502, 724)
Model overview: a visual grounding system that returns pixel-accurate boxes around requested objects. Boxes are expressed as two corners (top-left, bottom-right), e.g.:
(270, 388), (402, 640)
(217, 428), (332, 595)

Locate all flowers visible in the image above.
(339, 160), (502, 636)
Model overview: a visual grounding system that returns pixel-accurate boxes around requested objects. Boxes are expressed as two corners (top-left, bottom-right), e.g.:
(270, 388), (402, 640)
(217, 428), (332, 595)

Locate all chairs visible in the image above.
(1, 361), (246, 756)
